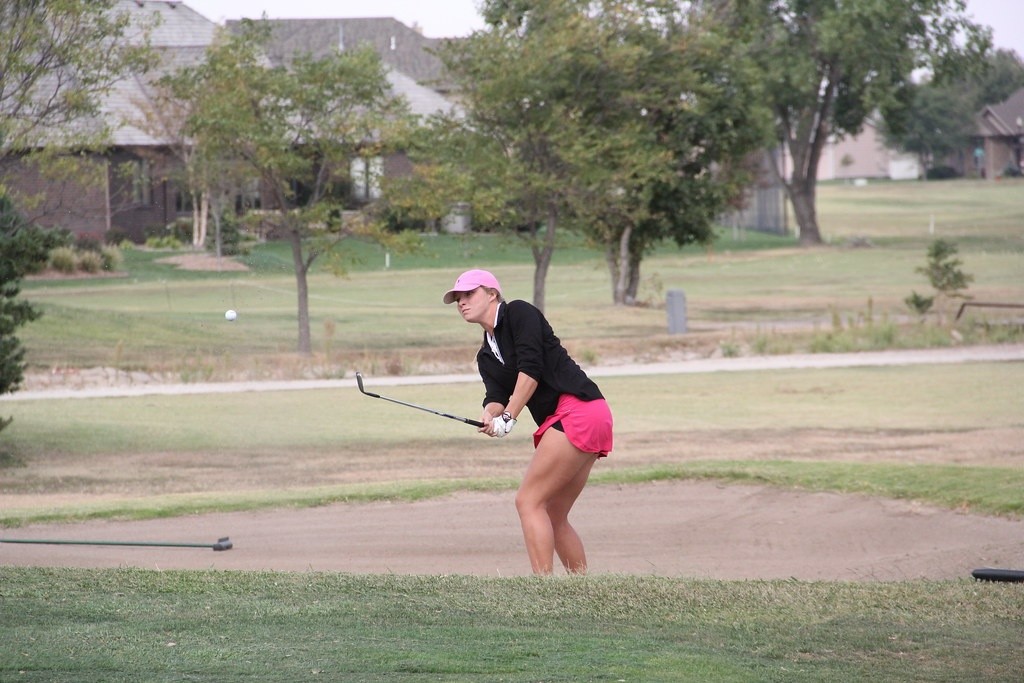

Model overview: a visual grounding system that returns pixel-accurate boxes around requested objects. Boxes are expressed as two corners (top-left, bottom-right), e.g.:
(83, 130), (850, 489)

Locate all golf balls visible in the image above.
(226, 310), (237, 320)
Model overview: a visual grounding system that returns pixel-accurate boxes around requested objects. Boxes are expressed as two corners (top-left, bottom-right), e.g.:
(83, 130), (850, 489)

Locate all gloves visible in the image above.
(492, 411), (517, 439)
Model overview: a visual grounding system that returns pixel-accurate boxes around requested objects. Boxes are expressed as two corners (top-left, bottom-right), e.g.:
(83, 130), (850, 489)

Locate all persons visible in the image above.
(443, 270), (614, 575)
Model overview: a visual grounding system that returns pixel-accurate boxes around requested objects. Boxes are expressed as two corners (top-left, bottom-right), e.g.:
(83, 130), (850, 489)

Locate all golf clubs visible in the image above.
(355, 371), (485, 429)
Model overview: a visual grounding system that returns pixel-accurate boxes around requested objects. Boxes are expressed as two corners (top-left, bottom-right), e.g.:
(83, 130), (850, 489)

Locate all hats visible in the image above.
(443, 269), (501, 304)
